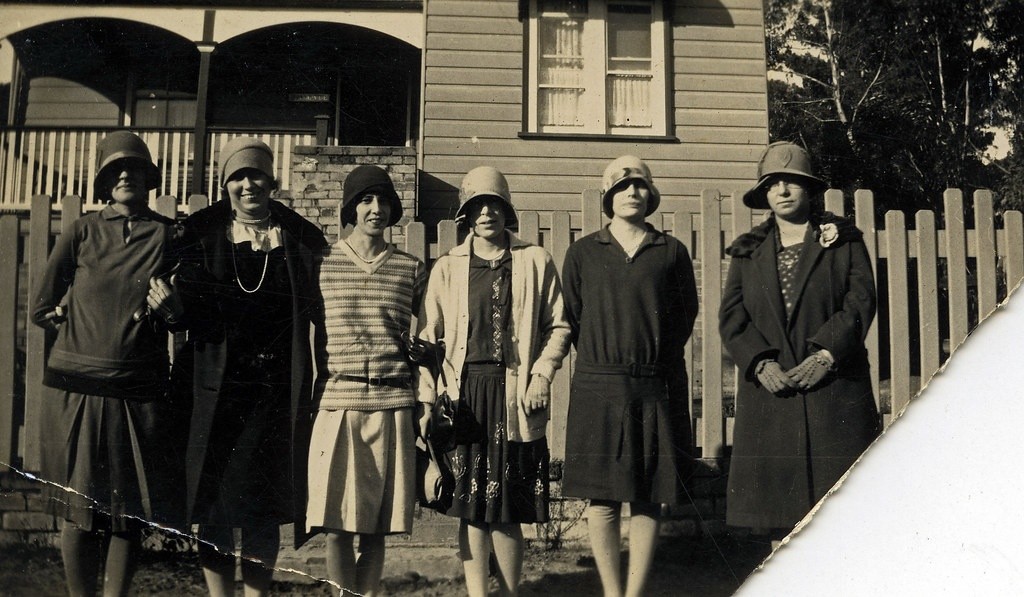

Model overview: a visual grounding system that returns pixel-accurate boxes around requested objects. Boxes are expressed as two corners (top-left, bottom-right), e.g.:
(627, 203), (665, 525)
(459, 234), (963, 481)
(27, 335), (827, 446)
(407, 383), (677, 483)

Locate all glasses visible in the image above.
(764, 176), (805, 189)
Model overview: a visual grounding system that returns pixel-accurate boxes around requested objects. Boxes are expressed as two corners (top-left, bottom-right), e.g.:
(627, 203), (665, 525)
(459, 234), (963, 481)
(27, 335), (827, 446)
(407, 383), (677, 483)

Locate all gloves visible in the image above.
(524, 374), (551, 417)
(145, 275), (186, 325)
(753, 358), (800, 397)
(785, 351), (834, 391)
(413, 402), (434, 440)
(405, 336), (446, 367)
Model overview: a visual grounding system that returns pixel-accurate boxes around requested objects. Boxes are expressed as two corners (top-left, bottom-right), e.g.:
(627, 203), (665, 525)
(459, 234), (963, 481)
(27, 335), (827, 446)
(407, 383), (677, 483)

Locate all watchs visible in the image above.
(164, 313), (182, 324)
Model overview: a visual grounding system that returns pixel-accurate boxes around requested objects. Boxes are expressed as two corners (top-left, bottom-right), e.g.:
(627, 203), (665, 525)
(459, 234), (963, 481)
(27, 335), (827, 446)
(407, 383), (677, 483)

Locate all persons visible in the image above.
(717, 141), (882, 552)
(170, 136), (328, 597)
(302, 165), (445, 597)
(32, 131), (200, 597)
(416, 166), (572, 597)
(561, 155), (699, 597)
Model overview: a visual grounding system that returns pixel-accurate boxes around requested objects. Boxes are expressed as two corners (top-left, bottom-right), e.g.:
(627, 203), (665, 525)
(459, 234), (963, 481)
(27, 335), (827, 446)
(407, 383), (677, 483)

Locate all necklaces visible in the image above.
(229, 210), (271, 293)
(489, 249), (505, 261)
(347, 236), (387, 264)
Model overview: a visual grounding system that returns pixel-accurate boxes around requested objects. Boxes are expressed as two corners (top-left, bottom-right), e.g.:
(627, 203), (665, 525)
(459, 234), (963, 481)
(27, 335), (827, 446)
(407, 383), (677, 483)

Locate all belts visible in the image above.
(317, 372), (412, 389)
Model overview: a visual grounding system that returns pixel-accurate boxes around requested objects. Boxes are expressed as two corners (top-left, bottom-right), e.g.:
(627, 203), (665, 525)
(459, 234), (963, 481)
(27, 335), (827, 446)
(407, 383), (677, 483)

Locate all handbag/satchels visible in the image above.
(412, 436), (444, 505)
(431, 391), (458, 445)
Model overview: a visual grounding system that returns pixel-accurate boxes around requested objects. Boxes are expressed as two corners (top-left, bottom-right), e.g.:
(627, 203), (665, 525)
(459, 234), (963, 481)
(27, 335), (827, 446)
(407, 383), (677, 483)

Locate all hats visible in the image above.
(744, 141), (832, 210)
(220, 138), (274, 188)
(454, 165), (520, 233)
(93, 131), (162, 200)
(340, 164), (403, 228)
(601, 156), (661, 219)
